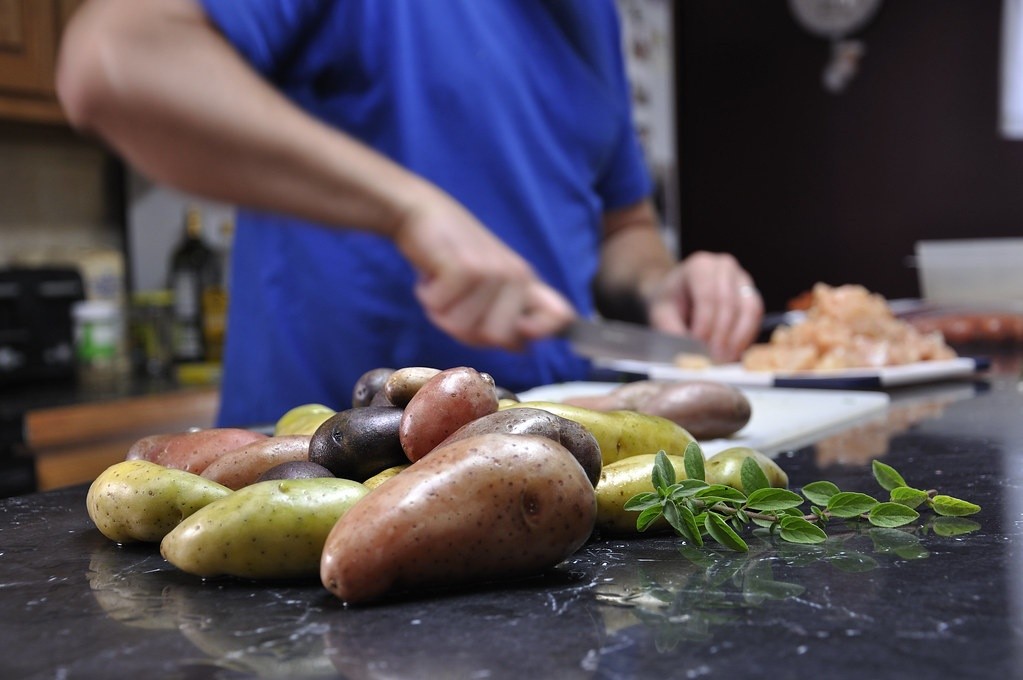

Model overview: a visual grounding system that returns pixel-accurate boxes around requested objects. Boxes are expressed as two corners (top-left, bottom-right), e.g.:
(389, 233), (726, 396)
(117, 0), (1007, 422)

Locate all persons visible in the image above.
(57, 0), (766, 428)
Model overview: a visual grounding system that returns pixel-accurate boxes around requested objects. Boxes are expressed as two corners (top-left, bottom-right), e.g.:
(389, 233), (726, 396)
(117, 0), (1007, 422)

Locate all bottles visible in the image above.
(163, 201), (231, 358)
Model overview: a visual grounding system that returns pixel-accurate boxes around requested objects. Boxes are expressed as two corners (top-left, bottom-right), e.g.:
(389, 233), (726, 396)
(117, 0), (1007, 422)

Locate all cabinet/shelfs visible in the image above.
(0, 0), (87, 124)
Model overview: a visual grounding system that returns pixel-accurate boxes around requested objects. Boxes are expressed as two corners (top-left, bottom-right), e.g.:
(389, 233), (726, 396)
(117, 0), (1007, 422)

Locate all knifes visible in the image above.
(556, 317), (710, 367)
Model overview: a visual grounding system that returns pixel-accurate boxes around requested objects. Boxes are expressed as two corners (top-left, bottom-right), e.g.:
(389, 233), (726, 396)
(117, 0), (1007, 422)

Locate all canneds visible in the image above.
(73, 289), (176, 382)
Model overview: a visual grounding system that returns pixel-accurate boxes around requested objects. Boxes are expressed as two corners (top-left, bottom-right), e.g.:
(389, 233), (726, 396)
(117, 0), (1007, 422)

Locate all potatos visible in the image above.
(84, 365), (787, 603)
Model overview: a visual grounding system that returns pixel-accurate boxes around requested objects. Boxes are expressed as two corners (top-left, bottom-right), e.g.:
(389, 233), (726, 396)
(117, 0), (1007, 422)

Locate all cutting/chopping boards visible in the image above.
(517, 378), (888, 462)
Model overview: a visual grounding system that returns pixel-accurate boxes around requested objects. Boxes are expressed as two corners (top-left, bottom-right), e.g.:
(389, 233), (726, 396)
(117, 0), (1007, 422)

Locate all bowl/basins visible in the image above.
(916, 239), (1023, 317)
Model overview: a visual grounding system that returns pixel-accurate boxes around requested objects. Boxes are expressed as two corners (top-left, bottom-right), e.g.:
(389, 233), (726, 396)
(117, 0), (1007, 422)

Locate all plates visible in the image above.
(656, 356), (993, 387)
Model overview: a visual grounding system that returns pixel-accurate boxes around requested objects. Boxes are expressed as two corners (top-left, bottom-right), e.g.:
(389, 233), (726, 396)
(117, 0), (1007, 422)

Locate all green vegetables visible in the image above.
(625, 443), (980, 545)
